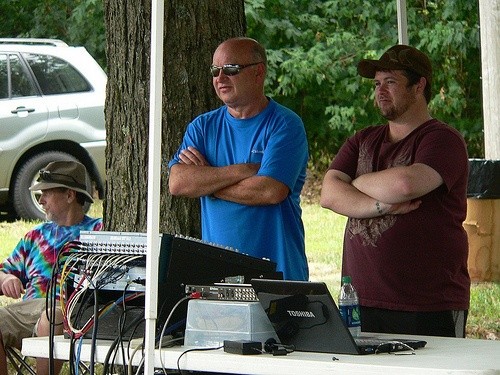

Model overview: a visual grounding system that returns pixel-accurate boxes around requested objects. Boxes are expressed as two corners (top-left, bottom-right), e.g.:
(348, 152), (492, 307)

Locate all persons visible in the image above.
(167, 37), (310, 283)
(320, 44), (470, 339)
(0, 160), (106, 375)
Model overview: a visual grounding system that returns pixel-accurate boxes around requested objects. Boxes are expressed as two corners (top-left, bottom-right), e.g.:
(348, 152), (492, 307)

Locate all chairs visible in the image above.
(5, 347), (91, 375)
(17, 64), (76, 95)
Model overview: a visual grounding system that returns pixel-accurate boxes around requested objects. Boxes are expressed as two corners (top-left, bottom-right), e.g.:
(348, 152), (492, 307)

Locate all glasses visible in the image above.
(39, 170), (84, 187)
(210, 61), (266, 77)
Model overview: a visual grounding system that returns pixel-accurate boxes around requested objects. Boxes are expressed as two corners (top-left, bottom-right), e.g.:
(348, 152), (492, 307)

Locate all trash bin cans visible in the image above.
(462, 159), (500, 281)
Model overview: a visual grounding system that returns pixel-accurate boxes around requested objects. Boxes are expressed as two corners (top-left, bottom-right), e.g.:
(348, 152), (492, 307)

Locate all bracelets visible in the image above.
(374, 200), (384, 216)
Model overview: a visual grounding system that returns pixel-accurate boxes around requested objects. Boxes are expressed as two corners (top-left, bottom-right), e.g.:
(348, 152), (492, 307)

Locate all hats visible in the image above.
(28, 161), (94, 203)
(357, 45), (435, 86)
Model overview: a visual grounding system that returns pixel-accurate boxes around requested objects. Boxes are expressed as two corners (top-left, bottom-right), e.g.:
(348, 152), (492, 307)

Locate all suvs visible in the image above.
(0, 36), (108, 223)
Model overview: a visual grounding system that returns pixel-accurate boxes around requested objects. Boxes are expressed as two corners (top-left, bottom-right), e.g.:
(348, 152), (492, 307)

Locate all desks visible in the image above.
(21, 332), (500, 375)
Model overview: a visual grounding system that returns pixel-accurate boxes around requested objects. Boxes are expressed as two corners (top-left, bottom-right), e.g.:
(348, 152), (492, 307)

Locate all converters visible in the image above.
(224, 340), (262, 355)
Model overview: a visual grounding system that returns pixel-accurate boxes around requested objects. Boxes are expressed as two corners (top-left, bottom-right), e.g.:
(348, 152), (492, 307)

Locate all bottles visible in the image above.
(338, 276), (361, 341)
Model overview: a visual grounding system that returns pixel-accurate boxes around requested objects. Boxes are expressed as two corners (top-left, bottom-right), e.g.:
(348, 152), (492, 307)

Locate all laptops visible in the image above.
(251, 279), (427, 355)
